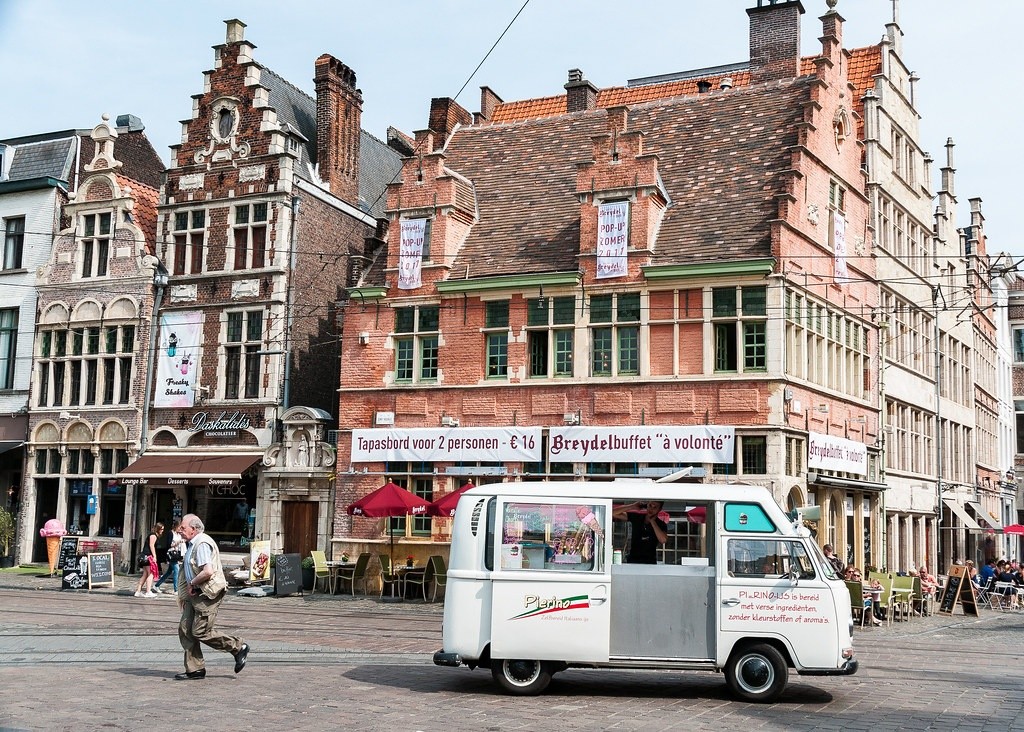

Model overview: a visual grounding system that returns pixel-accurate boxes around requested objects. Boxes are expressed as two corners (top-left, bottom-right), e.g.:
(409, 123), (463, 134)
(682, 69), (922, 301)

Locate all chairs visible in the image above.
(311, 550), (447, 603)
(843, 571), (1024, 629)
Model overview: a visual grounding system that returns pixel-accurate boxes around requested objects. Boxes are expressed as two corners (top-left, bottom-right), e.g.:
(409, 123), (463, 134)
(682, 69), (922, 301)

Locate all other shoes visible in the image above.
(145, 593), (157, 597)
(135, 590), (146, 597)
(154, 586), (163, 594)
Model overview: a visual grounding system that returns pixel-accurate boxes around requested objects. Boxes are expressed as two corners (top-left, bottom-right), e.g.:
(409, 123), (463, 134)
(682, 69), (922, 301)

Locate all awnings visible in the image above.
(968, 502), (1004, 534)
(118, 455), (263, 485)
(943, 499), (982, 535)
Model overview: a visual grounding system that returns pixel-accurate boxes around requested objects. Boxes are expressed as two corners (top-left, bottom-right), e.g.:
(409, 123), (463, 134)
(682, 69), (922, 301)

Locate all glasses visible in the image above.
(849, 571), (854, 573)
(920, 571), (925, 574)
(854, 574), (860, 577)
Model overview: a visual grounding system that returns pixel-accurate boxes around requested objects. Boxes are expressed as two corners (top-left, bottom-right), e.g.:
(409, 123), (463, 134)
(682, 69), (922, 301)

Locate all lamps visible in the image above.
(442, 417), (459, 427)
(192, 382), (211, 394)
(808, 403), (830, 413)
(564, 412), (580, 425)
(849, 414), (866, 423)
(60, 412), (81, 421)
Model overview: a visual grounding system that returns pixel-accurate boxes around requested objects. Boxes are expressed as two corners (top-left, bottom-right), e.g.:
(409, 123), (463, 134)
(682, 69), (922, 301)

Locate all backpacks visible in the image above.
(827, 557), (845, 580)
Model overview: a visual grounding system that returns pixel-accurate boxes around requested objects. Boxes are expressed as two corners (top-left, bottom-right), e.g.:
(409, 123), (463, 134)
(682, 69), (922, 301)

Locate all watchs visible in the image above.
(189, 581), (194, 587)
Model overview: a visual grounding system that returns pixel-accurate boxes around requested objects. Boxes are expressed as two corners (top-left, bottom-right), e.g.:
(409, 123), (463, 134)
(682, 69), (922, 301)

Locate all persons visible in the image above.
(965, 560), (978, 583)
(909, 565), (939, 612)
(845, 566), (885, 626)
(175, 514), (251, 680)
(823, 544), (836, 558)
(134, 522), (164, 598)
(613, 500), (668, 564)
(980, 557), (1024, 608)
(153, 520), (186, 595)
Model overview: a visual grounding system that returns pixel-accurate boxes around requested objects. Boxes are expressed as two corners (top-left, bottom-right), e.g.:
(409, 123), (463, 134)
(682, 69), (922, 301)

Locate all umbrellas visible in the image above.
(347, 478), (432, 597)
(613, 504), (670, 523)
(426, 479), (475, 517)
(687, 506), (706, 524)
(985, 523), (1024, 557)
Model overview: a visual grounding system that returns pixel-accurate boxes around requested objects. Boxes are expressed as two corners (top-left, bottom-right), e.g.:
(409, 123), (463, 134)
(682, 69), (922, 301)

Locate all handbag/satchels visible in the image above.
(137, 553), (148, 568)
(166, 550), (182, 563)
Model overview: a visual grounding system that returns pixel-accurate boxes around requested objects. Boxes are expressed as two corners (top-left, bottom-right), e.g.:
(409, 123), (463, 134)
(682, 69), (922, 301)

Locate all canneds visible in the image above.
(613, 550), (621, 565)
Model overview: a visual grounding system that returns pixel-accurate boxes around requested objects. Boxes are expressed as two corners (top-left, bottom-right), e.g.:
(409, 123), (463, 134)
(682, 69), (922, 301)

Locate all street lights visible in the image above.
(117, 255), (170, 578)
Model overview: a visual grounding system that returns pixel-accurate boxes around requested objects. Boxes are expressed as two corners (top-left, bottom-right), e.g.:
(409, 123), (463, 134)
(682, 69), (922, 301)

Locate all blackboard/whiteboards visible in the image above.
(62, 555), (89, 589)
(87, 552), (114, 584)
(58, 536), (79, 569)
(275, 553), (303, 594)
(939, 565), (978, 615)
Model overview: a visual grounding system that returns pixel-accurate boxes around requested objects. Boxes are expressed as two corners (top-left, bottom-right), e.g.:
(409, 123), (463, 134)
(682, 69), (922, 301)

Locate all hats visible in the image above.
(966, 560), (974, 566)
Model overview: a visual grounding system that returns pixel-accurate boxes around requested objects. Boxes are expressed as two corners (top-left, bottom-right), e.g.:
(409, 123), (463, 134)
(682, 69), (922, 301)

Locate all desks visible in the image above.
(320, 561), (357, 595)
(862, 589), (884, 627)
(891, 587), (914, 622)
(387, 566), (428, 600)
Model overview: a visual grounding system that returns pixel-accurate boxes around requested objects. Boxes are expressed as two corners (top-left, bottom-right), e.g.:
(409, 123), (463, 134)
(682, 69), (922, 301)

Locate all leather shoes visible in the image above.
(235, 643), (250, 673)
(175, 668), (206, 681)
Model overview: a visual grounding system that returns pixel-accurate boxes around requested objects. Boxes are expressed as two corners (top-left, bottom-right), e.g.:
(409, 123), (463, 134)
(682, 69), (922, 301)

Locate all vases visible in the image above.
(407, 559), (413, 567)
(342, 553), (349, 562)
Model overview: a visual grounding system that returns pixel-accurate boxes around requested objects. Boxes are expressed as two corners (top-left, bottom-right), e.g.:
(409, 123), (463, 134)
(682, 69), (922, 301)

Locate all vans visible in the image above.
(432, 465), (860, 703)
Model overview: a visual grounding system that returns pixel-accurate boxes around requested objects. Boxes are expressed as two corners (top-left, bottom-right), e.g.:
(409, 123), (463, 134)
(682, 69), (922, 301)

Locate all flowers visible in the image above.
(406, 554), (416, 560)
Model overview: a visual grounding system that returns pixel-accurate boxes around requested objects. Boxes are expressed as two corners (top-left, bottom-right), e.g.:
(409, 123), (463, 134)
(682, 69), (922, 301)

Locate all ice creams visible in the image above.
(39, 519), (67, 577)
(575, 506), (605, 538)
